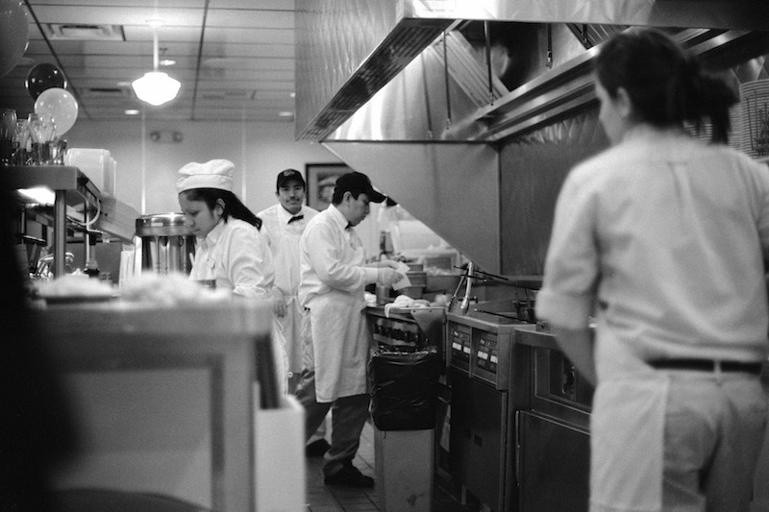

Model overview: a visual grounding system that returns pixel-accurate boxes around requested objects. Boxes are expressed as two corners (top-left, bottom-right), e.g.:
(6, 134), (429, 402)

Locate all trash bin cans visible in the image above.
(368, 344), (440, 512)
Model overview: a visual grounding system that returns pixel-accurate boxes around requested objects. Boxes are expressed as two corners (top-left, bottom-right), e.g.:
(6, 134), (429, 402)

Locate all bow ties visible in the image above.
(288, 215), (303, 224)
(345, 221), (351, 229)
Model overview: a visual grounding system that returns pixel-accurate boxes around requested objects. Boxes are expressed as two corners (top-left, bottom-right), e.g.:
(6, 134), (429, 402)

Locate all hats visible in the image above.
(277, 169), (305, 192)
(335, 171), (387, 203)
(176, 159), (237, 194)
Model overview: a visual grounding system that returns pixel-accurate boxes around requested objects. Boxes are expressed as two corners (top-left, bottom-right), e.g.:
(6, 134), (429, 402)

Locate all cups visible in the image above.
(0, 108), (56, 167)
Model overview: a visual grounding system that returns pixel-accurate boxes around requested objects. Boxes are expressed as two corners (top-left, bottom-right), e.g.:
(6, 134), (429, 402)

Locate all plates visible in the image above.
(684, 78), (769, 161)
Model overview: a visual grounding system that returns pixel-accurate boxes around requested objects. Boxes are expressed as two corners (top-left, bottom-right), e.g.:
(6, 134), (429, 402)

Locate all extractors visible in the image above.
(292, 0), (769, 142)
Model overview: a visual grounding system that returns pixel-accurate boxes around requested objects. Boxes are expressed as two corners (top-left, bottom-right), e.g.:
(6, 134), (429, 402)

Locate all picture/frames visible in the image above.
(304, 162), (357, 213)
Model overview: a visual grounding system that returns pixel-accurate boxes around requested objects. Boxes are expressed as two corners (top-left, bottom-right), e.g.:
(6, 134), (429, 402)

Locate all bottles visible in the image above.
(376, 284), (388, 305)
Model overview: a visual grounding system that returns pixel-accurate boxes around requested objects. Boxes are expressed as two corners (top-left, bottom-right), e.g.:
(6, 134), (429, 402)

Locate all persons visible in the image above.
(173, 159), (278, 300)
(531, 26), (769, 511)
(255, 169), (331, 458)
(296, 170), (405, 490)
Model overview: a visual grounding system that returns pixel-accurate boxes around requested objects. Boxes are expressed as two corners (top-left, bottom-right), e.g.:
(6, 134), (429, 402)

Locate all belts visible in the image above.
(645, 358), (763, 374)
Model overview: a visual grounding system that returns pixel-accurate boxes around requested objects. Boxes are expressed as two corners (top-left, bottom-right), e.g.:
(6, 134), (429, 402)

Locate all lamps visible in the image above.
(124, 16), (186, 108)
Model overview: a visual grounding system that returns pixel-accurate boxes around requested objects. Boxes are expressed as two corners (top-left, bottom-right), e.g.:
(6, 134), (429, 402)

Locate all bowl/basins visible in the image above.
(425, 274), (460, 293)
